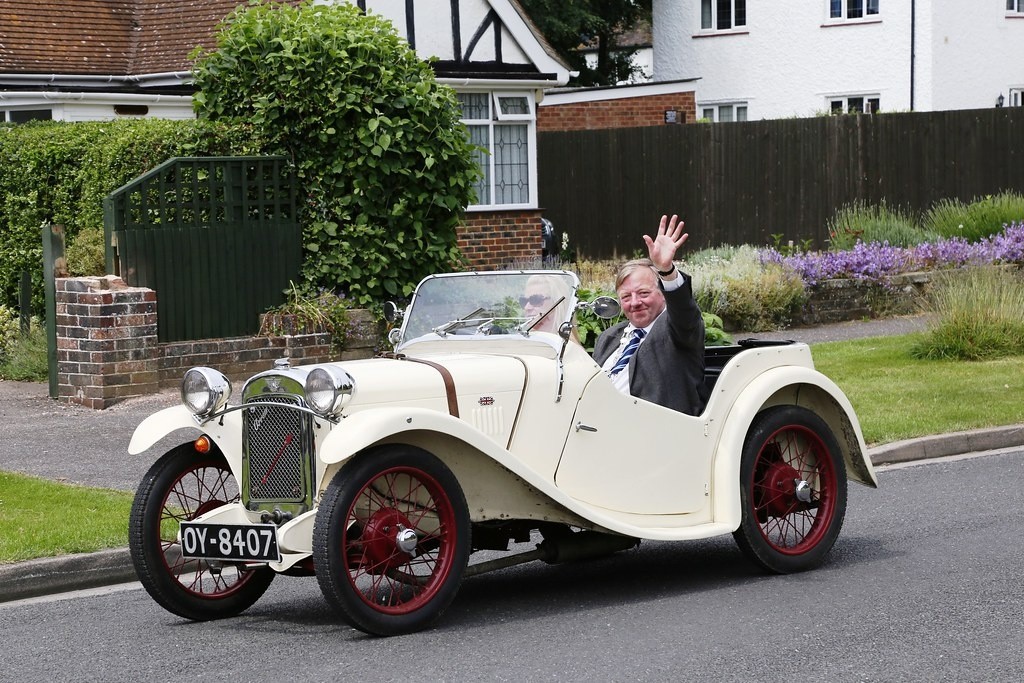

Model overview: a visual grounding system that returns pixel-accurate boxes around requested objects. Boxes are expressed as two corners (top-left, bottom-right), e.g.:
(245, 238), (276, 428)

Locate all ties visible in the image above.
(611, 329), (647, 374)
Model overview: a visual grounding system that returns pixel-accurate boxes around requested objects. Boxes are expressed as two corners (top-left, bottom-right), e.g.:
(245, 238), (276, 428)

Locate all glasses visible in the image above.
(519, 294), (551, 309)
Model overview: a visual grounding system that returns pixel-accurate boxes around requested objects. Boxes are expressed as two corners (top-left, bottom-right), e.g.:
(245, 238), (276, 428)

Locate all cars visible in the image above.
(125, 263), (879, 643)
(541, 218), (557, 260)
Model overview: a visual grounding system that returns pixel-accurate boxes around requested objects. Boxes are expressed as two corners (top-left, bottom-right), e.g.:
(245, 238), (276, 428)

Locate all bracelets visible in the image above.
(656, 262), (676, 276)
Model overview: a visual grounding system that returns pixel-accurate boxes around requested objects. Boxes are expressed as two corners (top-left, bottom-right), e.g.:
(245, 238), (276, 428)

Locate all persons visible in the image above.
(592, 215), (706, 417)
(519, 273), (582, 347)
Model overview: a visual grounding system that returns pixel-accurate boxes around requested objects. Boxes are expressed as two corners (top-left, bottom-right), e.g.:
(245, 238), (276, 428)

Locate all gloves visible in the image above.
(490, 325), (509, 334)
